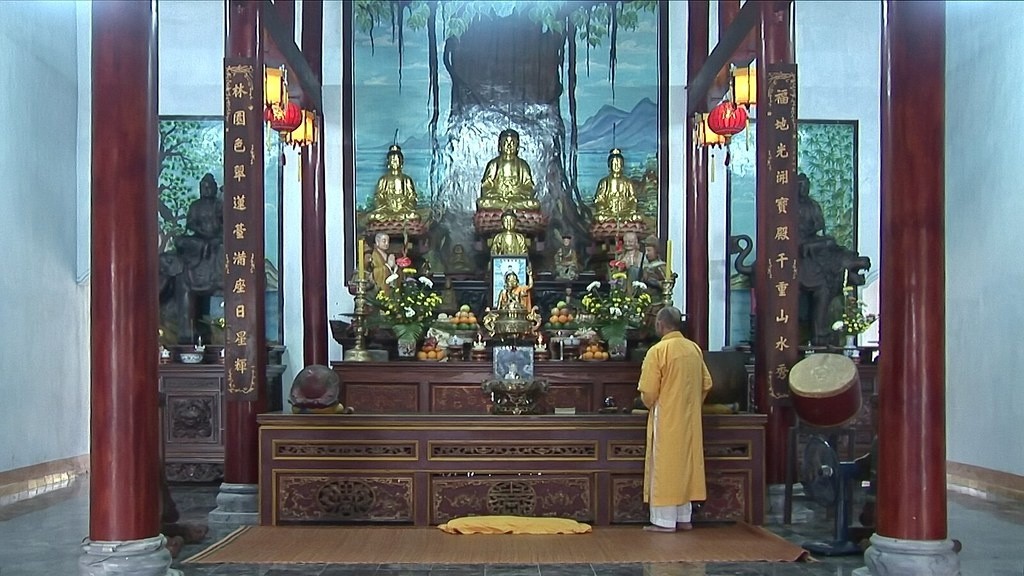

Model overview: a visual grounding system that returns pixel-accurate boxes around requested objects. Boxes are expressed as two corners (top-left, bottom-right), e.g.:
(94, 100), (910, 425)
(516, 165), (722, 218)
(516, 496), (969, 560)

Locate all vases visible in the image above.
(397, 339), (419, 359)
(845, 331), (860, 359)
(608, 340), (629, 360)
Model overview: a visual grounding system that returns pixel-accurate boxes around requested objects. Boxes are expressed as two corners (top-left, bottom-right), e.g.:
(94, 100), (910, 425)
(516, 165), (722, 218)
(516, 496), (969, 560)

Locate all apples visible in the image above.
(551, 301), (568, 316)
(455, 304), (475, 318)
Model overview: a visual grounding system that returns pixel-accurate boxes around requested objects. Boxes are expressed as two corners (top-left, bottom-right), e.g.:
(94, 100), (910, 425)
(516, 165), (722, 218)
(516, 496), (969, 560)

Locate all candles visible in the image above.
(358, 239), (363, 281)
(665, 239), (672, 280)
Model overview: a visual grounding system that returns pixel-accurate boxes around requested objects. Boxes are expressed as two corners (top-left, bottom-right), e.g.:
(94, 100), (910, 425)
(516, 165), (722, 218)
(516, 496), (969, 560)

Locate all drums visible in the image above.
(789, 353), (862, 429)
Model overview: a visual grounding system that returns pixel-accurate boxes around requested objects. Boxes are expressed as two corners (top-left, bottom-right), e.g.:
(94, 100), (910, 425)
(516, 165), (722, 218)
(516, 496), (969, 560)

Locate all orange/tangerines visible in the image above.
(582, 346), (608, 360)
(549, 314), (573, 323)
(451, 316), (477, 324)
(418, 346), (445, 360)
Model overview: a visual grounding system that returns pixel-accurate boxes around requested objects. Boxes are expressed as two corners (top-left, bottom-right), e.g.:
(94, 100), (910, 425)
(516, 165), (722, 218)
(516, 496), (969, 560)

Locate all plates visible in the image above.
(583, 358), (603, 362)
(547, 329), (575, 336)
(454, 329), (474, 338)
(422, 359), (438, 362)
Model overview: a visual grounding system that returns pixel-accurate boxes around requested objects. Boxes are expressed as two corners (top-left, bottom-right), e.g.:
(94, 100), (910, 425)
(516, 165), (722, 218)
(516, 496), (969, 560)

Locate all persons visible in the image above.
(637, 307), (713, 531)
(174, 173), (224, 290)
(592, 149), (638, 224)
(476, 129), (541, 211)
(444, 244), (475, 275)
(371, 232), (403, 291)
(560, 287), (575, 303)
(798, 173), (825, 238)
(497, 267), (533, 309)
(367, 145), (420, 221)
(618, 233), (676, 326)
(554, 235), (579, 280)
(487, 211), (531, 274)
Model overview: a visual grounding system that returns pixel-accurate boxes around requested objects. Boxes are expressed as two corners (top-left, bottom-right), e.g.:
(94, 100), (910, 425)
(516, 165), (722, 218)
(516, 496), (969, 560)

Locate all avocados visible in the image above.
(543, 322), (579, 330)
(449, 323), (477, 330)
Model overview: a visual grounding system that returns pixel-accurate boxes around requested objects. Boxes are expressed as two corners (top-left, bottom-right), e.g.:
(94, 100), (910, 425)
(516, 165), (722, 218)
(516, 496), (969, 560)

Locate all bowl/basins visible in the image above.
(564, 339), (581, 345)
(180, 353), (203, 364)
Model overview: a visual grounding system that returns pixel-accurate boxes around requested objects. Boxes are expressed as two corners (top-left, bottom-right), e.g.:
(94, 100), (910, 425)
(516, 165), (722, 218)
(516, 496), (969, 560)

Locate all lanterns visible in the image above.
(264, 64), (318, 182)
(695, 68), (756, 182)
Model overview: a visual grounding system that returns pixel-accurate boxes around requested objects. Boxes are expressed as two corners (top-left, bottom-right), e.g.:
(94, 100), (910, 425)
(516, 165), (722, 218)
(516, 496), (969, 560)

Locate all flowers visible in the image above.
(371, 257), (439, 323)
(584, 263), (648, 321)
(831, 267), (880, 333)
